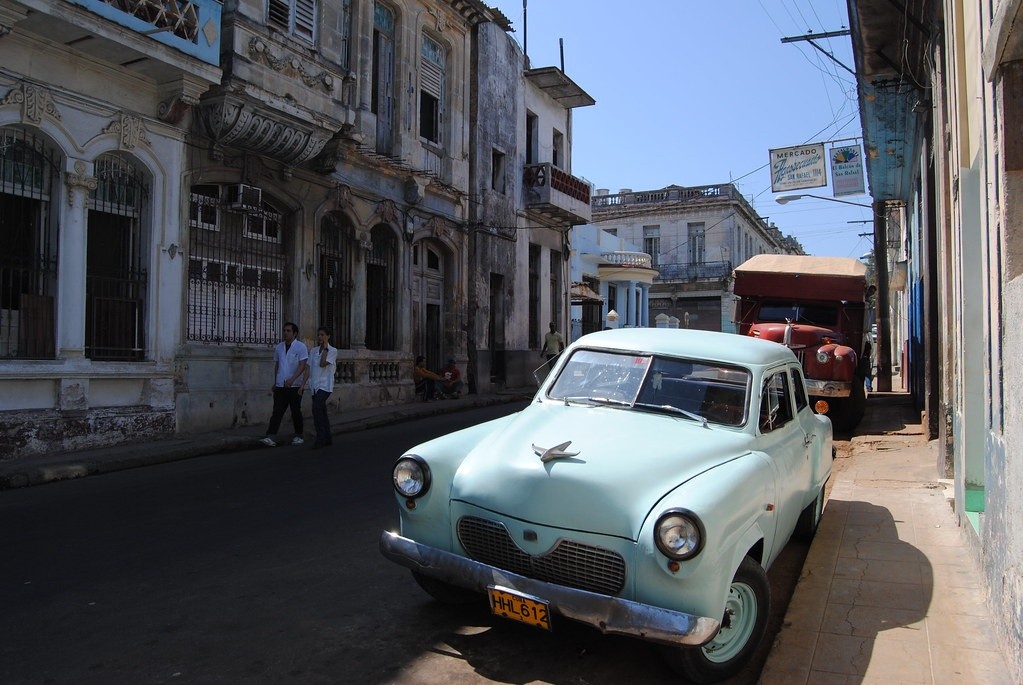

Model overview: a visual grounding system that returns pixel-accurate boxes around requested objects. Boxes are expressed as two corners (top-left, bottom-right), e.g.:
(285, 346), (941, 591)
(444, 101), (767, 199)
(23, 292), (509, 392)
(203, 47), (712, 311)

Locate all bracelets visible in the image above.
(324, 347), (329, 352)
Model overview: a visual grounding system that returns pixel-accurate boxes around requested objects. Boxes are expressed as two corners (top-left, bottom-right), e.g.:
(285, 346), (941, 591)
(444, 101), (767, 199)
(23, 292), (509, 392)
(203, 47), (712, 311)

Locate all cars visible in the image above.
(375, 323), (838, 682)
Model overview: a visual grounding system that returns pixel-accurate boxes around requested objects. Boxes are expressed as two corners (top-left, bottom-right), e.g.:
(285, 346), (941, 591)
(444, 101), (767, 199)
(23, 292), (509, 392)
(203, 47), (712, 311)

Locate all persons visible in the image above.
(437, 360), (463, 399)
(298, 328), (339, 448)
(415, 356), (446, 402)
(540, 321), (564, 372)
(860, 330), (876, 393)
(261, 322), (308, 446)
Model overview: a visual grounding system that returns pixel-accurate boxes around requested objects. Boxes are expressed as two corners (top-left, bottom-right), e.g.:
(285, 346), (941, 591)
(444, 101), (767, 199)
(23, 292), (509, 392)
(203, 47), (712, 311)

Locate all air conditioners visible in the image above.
(228, 182), (263, 209)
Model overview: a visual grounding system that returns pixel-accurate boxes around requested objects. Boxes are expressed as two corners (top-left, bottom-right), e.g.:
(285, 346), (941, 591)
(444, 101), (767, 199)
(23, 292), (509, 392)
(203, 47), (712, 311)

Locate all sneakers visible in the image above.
(291, 436), (304, 446)
(258, 437), (276, 447)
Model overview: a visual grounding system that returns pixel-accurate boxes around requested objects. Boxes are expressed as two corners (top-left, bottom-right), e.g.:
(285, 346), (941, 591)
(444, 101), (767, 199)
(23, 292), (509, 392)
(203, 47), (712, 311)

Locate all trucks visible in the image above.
(720, 254), (878, 424)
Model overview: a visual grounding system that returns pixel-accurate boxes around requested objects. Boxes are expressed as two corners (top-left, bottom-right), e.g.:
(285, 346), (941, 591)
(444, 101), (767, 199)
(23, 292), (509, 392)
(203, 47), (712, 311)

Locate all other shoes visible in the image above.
(439, 392), (447, 400)
(424, 398), (431, 403)
(450, 392), (459, 399)
(430, 396), (438, 400)
(312, 440), (332, 450)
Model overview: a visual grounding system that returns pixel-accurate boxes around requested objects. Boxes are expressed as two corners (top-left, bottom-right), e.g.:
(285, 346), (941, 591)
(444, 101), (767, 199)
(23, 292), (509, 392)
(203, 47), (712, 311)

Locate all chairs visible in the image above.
(705, 401), (766, 433)
